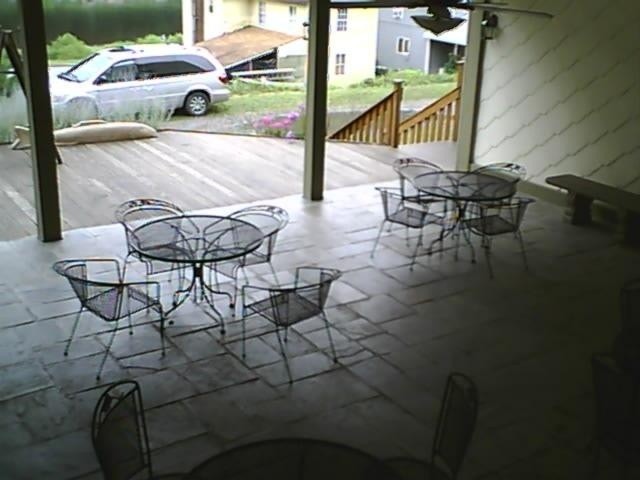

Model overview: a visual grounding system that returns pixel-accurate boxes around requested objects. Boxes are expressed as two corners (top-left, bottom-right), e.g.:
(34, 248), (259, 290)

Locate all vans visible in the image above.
(47, 39), (235, 127)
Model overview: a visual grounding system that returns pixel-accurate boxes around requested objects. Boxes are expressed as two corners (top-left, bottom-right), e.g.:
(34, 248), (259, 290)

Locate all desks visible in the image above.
(412, 169), (517, 265)
(129, 213), (266, 335)
(188, 437), (403, 480)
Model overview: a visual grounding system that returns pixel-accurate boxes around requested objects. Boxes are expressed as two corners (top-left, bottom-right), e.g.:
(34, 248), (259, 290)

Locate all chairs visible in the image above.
(52, 256), (168, 380)
(381, 369), (481, 478)
(453, 196), (538, 280)
(88, 379), (189, 480)
(114, 198), (202, 299)
(197, 204), (291, 318)
(238, 263), (346, 384)
(392, 156), (455, 243)
(450, 161), (528, 257)
(369, 185), (445, 269)
(581, 275), (639, 480)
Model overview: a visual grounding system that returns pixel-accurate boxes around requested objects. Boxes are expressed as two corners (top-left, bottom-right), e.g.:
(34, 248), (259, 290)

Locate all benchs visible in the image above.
(544, 172), (639, 245)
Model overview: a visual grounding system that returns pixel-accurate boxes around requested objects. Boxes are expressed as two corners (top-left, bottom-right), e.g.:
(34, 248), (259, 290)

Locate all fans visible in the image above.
(324, 1), (555, 21)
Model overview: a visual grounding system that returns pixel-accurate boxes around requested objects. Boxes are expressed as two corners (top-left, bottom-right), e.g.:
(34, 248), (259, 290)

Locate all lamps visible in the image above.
(477, 12), (502, 41)
(409, 15), (466, 36)
